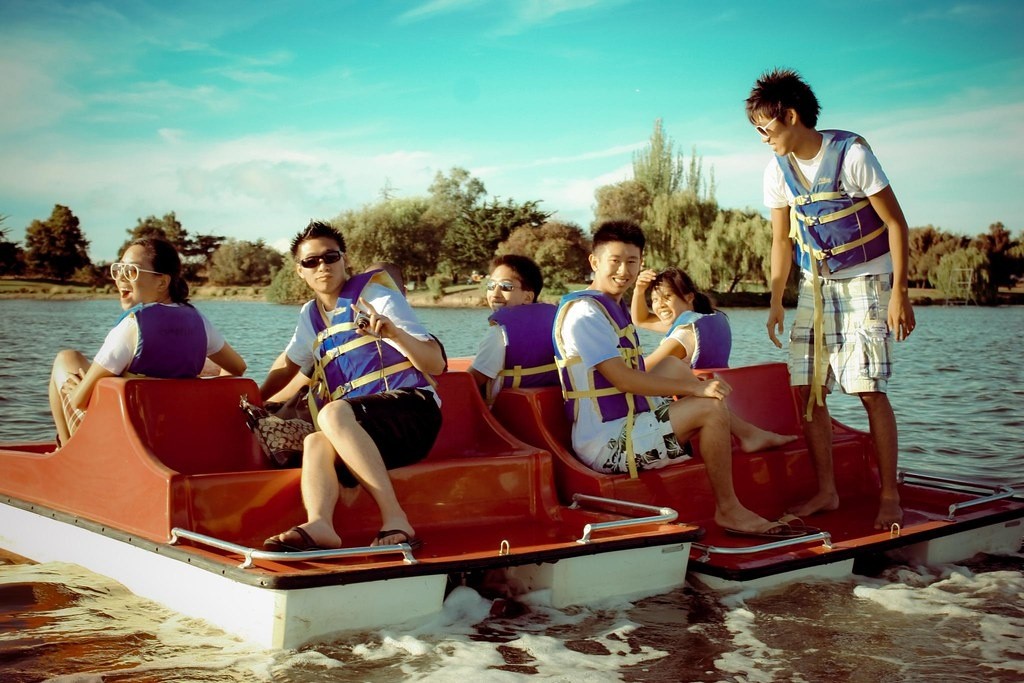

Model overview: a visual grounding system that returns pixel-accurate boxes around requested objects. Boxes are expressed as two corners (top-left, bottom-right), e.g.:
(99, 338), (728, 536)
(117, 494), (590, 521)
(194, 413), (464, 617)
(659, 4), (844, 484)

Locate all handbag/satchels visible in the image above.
(240, 394), (316, 469)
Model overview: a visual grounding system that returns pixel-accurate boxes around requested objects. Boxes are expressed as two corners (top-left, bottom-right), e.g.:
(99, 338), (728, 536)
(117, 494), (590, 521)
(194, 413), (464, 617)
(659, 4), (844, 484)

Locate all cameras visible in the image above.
(353, 311), (382, 337)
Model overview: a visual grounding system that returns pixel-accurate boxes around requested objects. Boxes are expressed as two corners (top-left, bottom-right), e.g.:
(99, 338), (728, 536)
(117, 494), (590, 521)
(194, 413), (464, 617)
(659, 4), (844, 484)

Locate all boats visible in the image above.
(437, 354), (1024, 596)
(1, 357), (709, 657)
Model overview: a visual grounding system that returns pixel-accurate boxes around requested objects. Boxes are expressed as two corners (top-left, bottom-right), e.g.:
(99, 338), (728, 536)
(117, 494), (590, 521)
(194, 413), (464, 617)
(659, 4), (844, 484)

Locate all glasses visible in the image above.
(486, 281), (521, 292)
(110, 263), (163, 282)
(297, 250), (343, 268)
(755, 117), (777, 138)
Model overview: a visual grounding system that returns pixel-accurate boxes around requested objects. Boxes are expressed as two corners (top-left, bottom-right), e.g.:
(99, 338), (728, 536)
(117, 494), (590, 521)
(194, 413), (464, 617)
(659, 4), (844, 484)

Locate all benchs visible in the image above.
(50, 362), (882, 540)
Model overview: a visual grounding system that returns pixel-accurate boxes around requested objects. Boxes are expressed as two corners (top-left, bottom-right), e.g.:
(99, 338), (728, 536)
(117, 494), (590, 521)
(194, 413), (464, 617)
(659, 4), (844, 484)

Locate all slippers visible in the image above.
(263, 526), (335, 552)
(725, 512), (821, 540)
(374, 529), (422, 556)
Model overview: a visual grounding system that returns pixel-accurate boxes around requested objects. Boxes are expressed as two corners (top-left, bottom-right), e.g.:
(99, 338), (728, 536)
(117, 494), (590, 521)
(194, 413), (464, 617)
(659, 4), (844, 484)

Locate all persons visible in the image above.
(260, 219), (447, 559)
(551, 219), (798, 534)
(746, 68), (916, 531)
(468, 254), (559, 409)
(45, 238), (247, 455)
